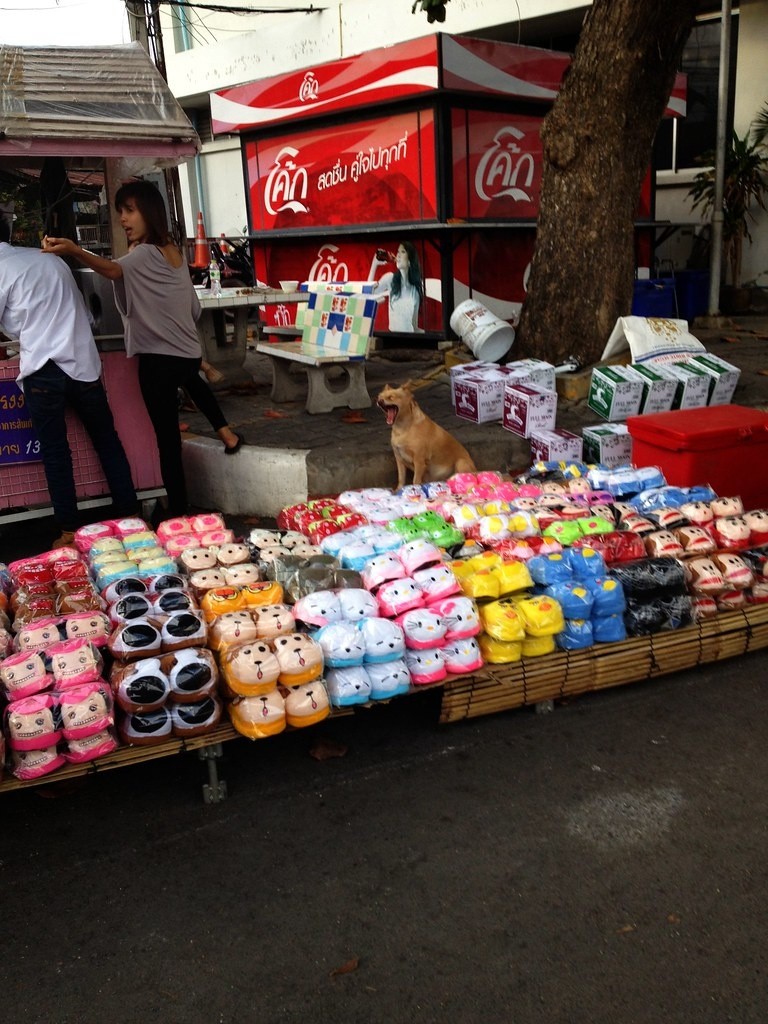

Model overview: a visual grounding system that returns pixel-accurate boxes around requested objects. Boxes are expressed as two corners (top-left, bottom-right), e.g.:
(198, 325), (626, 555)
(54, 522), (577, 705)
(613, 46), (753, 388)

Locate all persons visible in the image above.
(41, 178), (245, 514)
(0, 236), (139, 550)
(367, 243), (426, 334)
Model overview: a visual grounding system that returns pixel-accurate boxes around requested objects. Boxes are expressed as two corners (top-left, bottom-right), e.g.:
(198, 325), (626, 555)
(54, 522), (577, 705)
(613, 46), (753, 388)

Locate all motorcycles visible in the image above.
(187, 226), (257, 322)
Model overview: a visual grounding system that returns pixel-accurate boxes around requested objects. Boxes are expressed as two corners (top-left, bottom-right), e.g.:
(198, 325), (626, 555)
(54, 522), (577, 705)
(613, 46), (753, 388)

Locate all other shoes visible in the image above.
(52, 530), (75, 550)
(206, 368), (223, 384)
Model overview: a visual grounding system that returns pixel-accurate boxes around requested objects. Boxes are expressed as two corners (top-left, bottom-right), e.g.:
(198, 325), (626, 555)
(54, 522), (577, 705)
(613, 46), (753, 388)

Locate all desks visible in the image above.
(195, 286), (312, 391)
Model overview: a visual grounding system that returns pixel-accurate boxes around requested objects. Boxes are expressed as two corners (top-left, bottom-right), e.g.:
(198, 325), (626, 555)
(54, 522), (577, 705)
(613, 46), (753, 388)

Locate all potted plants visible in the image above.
(683, 127), (768, 314)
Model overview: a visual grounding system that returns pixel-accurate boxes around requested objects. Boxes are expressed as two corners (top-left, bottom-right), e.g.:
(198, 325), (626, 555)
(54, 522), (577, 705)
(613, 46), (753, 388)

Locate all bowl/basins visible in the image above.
(278, 280), (299, 294)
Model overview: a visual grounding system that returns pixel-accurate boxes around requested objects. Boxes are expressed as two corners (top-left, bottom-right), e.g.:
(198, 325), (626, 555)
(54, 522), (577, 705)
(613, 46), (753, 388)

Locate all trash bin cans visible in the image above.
(632, 277), (675, 318)
(674, 269), (708, 327)
(626, 404), (768, 512)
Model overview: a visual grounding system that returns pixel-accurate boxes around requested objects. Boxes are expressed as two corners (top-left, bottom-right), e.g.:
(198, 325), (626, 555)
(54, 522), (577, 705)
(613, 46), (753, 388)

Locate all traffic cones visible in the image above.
(189, 210), (212, 269)
(217, 233), (234, 274)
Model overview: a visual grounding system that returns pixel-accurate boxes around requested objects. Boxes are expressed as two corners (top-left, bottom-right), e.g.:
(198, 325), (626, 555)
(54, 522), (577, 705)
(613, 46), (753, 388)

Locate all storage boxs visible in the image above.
(449, 352), (768, 515)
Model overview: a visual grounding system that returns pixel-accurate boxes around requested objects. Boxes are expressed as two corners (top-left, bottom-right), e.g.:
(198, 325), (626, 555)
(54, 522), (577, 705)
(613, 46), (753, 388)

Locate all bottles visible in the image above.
(209, 260), (222, 296)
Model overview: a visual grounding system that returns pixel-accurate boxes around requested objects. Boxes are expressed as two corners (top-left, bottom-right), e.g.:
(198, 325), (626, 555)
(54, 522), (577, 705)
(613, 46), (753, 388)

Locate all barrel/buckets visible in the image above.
(449, 299), (515, 364)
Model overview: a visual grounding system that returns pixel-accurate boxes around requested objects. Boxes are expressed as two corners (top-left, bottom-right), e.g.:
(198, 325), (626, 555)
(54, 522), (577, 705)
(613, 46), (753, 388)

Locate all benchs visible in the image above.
(256, 282), (387, 415)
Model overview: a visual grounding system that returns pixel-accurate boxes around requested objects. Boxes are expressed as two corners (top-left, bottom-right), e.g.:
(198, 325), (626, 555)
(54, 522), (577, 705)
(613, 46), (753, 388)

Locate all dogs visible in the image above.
(377, 379), (476, 495)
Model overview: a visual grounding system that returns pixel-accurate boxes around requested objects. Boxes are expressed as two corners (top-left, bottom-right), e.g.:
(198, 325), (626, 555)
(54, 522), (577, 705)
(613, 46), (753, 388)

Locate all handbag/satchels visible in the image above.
(211, 237), (257, 288)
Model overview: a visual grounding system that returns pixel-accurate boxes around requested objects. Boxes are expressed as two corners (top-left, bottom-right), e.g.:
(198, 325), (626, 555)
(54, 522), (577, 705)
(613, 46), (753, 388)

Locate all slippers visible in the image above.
(225, 432), (244, 454)
(0, 462), (768, 779)
